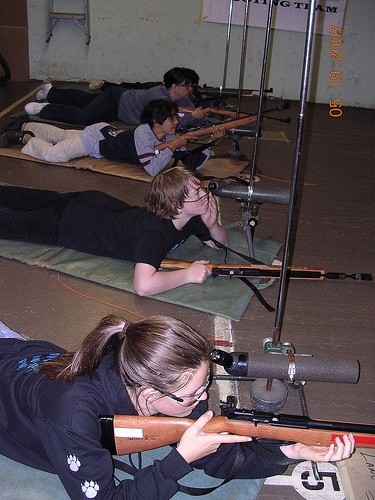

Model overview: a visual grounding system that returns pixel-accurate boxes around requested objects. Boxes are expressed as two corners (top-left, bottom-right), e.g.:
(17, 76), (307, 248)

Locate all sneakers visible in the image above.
(1, 121), (23, 133)
(25, 103), (50, 116)
(89, 80), (106, 91)
(0, 130), (34, 146)
(111, 78), (123, 84)
(36, 83), (52, 101)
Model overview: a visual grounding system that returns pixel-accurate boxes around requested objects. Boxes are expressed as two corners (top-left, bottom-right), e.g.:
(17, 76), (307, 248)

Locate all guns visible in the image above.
(98, 394), (375, 456)
(160, 257), (373, 282)
(196, 83), (273, 99)
(177, 106), (291, 125)
(155, 105), (290, 152)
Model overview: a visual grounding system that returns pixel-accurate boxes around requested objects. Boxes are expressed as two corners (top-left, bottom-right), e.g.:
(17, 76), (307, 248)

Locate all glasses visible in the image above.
(182, 188), (210, 208)
(127, 368), (211, 408)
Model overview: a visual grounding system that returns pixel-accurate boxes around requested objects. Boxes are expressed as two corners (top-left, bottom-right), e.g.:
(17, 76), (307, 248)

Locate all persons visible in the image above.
(0, 166), (230, 296)
(0, 314), (355, 500)
(0, 98), (226, 177)
(24, 66), (234, 127)
